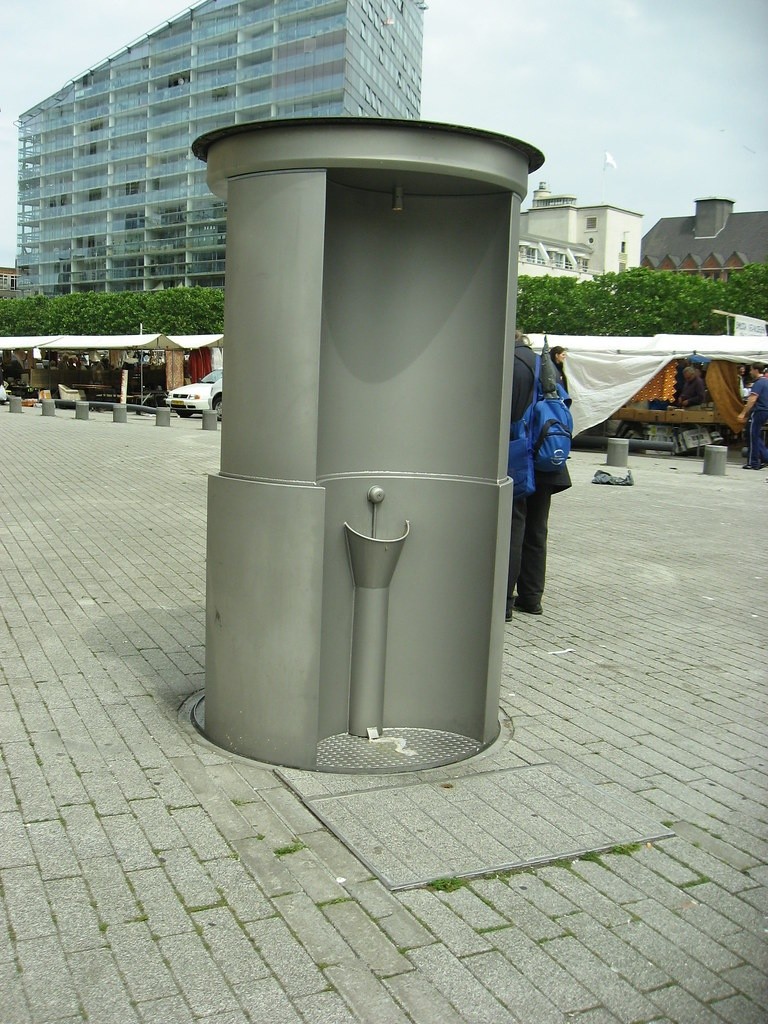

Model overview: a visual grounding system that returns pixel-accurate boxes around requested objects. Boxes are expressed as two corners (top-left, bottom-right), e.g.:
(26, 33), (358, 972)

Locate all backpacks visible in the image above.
(514, 353), (574, 472)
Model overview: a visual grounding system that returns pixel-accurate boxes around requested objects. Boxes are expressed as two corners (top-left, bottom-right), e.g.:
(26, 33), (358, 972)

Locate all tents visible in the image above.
(525, 333), (768, 437)
(0, 333), (225, 405)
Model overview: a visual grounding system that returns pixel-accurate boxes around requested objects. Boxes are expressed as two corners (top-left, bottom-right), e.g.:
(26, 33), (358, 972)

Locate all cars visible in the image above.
(165, 365), (222, 418)
(208, 376), (222, 421)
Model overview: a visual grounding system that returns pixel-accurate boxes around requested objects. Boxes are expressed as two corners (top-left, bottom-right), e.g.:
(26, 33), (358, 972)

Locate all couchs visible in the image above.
(57, 384), (86, 400)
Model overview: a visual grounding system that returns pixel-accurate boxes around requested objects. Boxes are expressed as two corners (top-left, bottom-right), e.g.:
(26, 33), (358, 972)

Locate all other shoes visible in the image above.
(742, 464), (754, 469)
(759, 462), (768, 469)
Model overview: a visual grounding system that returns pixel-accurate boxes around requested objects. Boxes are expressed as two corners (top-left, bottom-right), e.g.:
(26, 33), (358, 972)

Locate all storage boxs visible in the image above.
(611, 407), (723, 455)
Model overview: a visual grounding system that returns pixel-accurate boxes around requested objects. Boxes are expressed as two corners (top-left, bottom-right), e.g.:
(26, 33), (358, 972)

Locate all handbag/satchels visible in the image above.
(509, 436), (536, 501)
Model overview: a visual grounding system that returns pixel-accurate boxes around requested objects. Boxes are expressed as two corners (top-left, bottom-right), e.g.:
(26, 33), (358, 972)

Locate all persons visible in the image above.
(737, 365), (745, 397)
(551, 346), (571, 459)
(7, 348), (30, 378)
(737, 362), (768, 469)
(678, 367), (706, 407)
(505, 330), (572, 621)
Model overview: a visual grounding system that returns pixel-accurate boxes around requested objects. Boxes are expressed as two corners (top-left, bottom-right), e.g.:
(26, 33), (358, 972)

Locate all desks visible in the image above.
(614, 420), (718, 458)
(71, 384), (112, 401)
